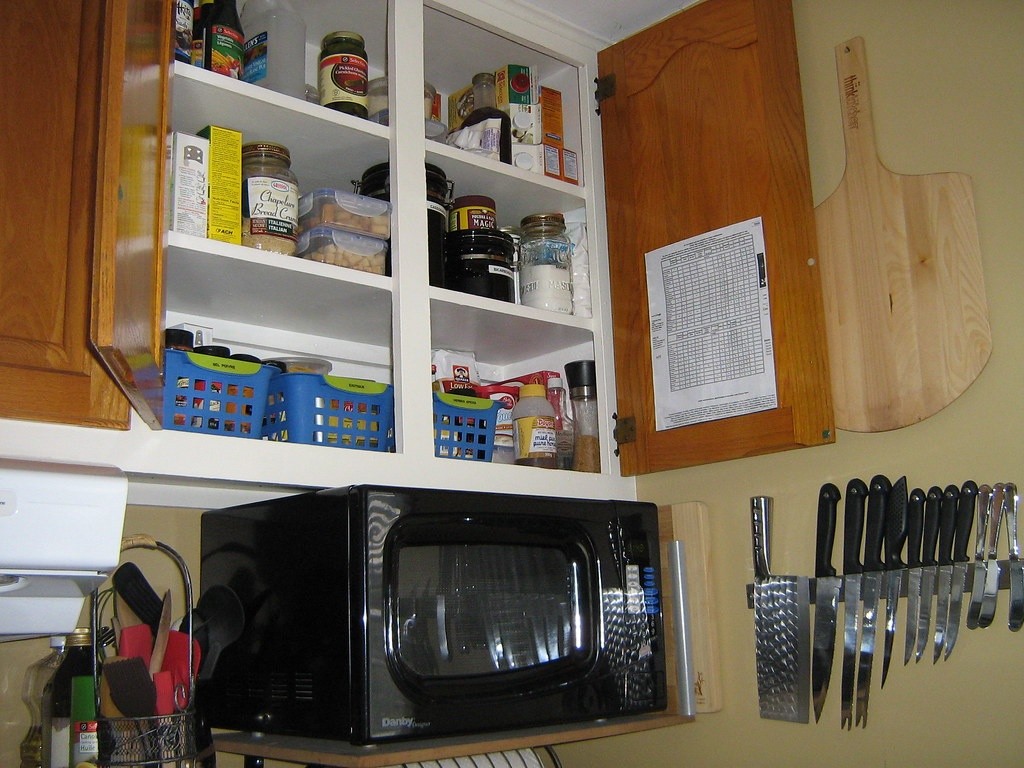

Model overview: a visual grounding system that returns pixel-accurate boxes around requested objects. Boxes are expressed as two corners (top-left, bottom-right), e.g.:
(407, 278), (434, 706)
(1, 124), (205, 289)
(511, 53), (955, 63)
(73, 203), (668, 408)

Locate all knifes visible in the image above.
(749, 495), (809, 724)
(813, 475), (1024, 729)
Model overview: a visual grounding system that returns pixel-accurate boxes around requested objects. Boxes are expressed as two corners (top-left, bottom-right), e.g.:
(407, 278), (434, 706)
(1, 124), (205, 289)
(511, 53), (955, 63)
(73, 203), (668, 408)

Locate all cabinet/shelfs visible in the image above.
(0, 0), (836, 462)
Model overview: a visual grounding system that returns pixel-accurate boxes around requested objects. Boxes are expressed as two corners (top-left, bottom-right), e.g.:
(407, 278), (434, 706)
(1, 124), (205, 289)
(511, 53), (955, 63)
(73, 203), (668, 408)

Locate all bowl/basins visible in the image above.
(366, 75), (437, 118)
(368, 108), (448, 145)
(261, 355), (332, 374)
(296, 187), (392, 279)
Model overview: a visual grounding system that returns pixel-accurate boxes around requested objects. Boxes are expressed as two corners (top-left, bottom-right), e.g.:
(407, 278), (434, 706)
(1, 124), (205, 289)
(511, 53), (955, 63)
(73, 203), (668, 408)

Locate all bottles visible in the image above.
(450, 195), (497, 231)
(19, 636), (67, 768)
(446, 228), (515, 303)
(471, 73), (497, 109)
(511, 385), (558, 469)
(351, 160), (455, 286)
(518, 212), (575, 314)
(545, 378), (575, 470)
(162, 327), (286, 434)
(40, 628), (101, 768)
(240, 140), (297, 259)
(240, 0), (306, 103)
(175, 0), (244, 79)
(565, 360), (601, 474)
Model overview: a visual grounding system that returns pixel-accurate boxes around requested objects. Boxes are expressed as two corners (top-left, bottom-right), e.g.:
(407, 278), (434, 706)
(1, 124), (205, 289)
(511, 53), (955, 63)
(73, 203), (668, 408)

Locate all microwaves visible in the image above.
(200, 486), (667, 750)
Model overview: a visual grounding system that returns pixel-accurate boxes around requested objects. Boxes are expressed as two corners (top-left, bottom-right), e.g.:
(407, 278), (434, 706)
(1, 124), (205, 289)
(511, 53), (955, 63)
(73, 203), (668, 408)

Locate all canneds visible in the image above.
(498, 213), (572, 315)
(318, 31), (369, 120)
(241, 141), (299, 256)
(446, 195), (496, 230)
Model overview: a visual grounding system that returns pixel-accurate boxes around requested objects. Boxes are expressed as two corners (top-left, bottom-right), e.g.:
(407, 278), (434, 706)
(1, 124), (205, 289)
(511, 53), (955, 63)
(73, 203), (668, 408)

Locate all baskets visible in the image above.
(432, 390), (506, 463)
(262, 371), (393, 451)
(161, 349), (281, 437)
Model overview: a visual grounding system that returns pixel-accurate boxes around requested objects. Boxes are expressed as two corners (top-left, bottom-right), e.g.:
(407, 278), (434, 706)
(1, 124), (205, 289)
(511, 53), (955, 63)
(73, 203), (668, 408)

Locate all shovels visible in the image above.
(148, 588), (172, 683)
(113, 561), (164, 633)
(814, 36), (994, 432)
(103, 656), (162, 768)
